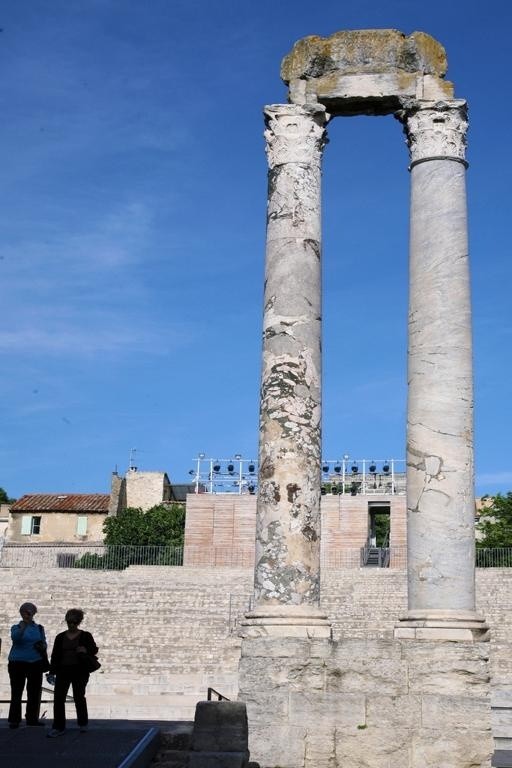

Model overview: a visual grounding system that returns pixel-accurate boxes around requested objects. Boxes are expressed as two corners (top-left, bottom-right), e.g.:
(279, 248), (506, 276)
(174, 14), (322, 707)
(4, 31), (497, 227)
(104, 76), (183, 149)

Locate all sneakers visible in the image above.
(80, 725), (88, 732)
(9, 723), (19, 729)
(47, 729), (65, 738)
(26, 721), (45, 726)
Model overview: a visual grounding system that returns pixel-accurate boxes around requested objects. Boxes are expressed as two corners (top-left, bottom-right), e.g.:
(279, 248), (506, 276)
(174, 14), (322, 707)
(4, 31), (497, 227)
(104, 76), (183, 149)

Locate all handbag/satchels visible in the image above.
(89, 655), (101, 672)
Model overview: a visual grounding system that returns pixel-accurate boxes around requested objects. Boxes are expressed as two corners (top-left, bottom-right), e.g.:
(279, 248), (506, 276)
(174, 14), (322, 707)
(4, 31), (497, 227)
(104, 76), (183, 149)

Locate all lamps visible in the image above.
(188, 452), (397, 494)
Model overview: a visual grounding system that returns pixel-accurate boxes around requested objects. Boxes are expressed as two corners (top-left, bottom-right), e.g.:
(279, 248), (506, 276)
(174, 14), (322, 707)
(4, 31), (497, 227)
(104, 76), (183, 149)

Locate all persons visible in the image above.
(8, 603), (49, 729)
(47, 610), (101, 738)
